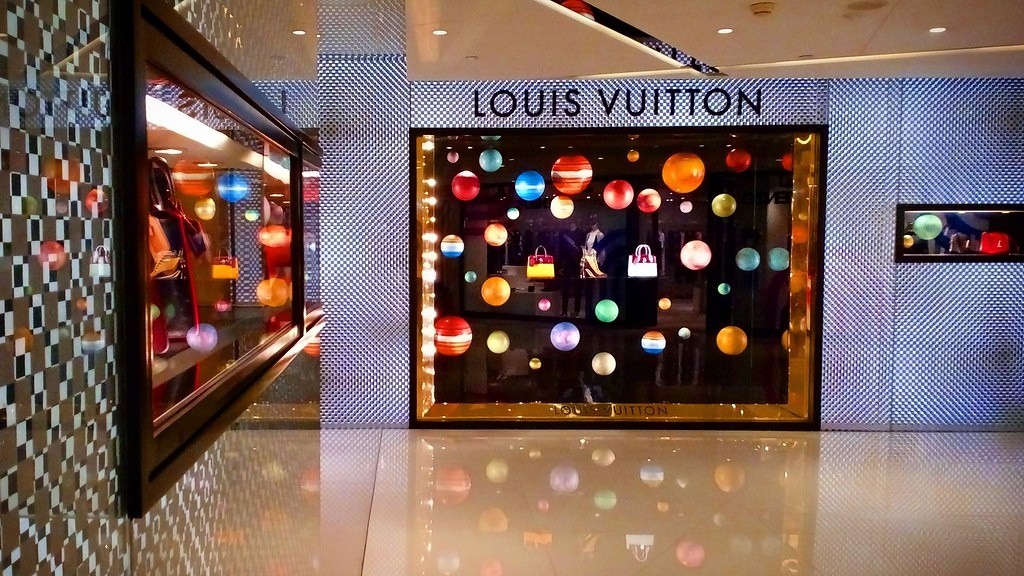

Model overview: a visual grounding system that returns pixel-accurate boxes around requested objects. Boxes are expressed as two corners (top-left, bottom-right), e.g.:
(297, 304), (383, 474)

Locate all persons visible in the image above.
(582, 214), (607, 319)
(555, 215), (585, 318)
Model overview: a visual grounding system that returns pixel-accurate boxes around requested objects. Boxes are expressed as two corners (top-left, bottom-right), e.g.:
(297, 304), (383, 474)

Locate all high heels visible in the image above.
(580, 247), (607, 277)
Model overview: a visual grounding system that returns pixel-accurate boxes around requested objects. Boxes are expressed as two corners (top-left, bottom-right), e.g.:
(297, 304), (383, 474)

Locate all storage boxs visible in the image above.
(585, 277), (658, 324)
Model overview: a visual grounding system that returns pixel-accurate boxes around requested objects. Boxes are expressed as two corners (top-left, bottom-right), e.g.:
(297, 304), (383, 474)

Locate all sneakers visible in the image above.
(559, 309), (580, 318)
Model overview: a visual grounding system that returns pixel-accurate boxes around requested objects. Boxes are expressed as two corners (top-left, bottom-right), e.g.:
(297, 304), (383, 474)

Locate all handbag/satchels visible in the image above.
(147, 157), (212, 278)
(213, 250), (239, 279)
(627, 246), (658, 276)
(527, 246), (554, 277)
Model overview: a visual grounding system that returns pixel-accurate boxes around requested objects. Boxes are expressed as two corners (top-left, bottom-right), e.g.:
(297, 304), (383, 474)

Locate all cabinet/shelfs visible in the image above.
(144, 91), (289, 391)
